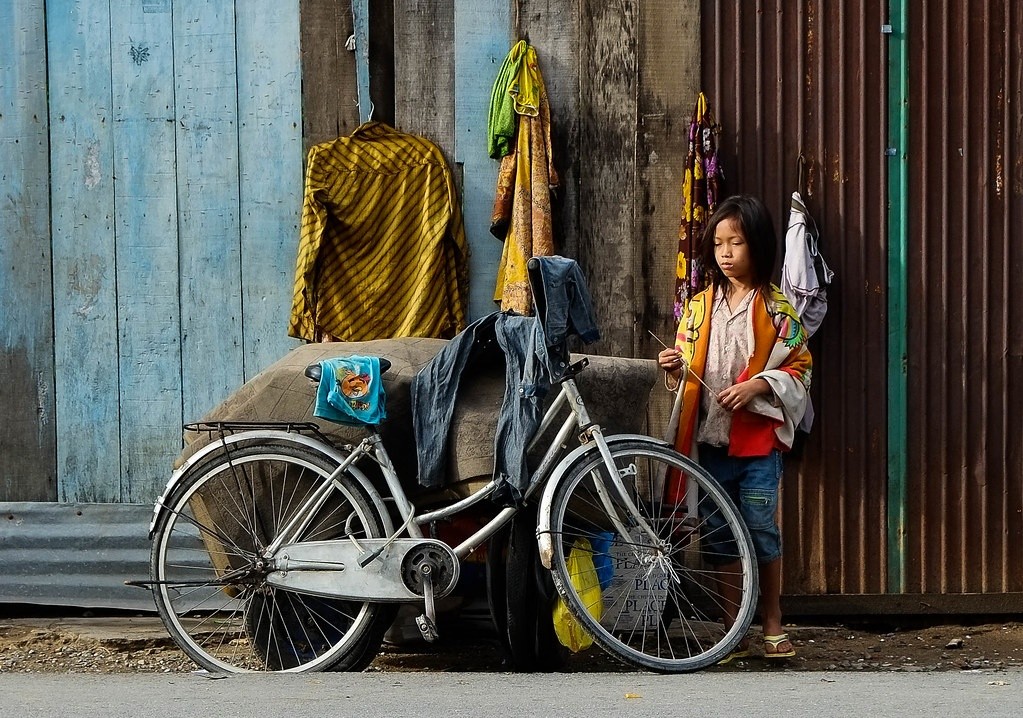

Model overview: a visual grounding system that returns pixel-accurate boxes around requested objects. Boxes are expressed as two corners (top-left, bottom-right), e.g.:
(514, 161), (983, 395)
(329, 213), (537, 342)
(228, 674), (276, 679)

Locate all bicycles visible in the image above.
(124, 257), (760, 678)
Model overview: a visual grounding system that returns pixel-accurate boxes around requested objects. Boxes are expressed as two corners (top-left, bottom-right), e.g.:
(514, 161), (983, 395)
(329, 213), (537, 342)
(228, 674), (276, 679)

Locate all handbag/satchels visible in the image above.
(552, 536), (602, 653)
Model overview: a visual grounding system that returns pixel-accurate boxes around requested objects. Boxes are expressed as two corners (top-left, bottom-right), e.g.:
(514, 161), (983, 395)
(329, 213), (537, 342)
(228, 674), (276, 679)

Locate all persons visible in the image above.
(658, 194), (812, 665)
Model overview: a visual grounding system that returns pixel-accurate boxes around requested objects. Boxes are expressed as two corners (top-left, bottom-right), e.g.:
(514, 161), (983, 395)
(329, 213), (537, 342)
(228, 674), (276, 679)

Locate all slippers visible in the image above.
(763, 633), (796, 658)
(715, 648), (756, 666)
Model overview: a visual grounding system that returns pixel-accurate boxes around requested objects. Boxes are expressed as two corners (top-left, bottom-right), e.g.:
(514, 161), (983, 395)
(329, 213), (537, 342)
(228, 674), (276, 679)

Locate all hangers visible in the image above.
(355, 100), (374, 121)
